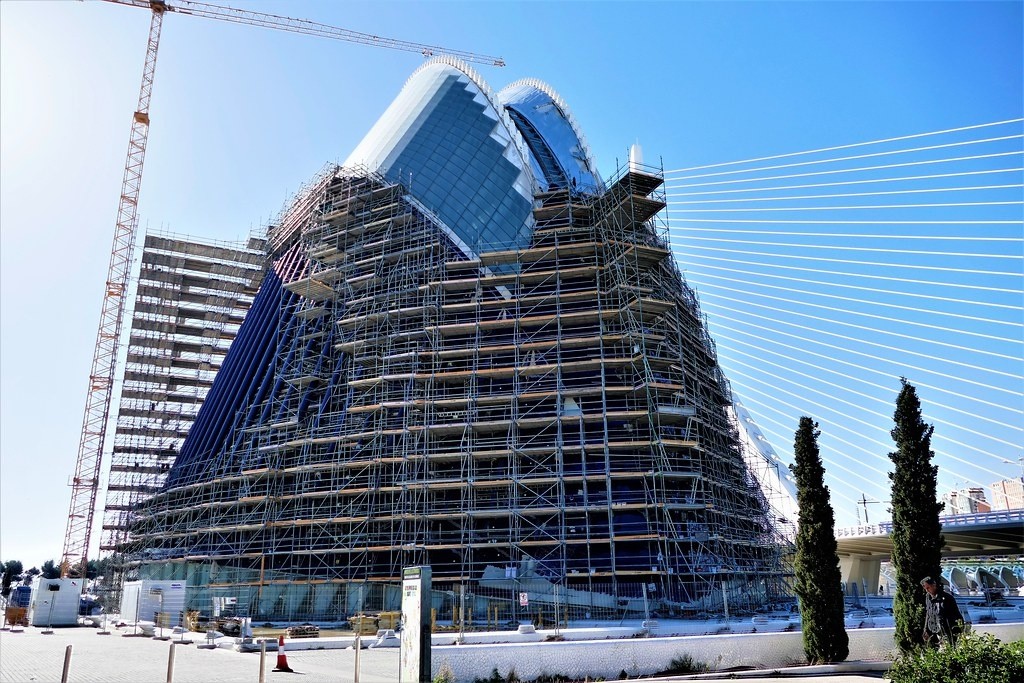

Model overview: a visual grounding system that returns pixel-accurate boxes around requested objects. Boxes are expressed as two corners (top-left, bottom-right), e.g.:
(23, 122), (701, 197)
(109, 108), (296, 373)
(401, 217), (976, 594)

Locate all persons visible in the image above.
(919, 576), (965, 648)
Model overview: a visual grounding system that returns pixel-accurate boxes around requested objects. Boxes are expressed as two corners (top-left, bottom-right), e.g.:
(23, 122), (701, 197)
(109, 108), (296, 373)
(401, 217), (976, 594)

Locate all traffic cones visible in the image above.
(270, 635), (294, 673)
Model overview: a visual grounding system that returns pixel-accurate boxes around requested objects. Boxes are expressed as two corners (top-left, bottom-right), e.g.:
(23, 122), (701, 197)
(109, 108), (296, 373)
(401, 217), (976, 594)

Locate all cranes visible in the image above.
(856, 493), (892, 523)
(56, 1), (509, 585)
